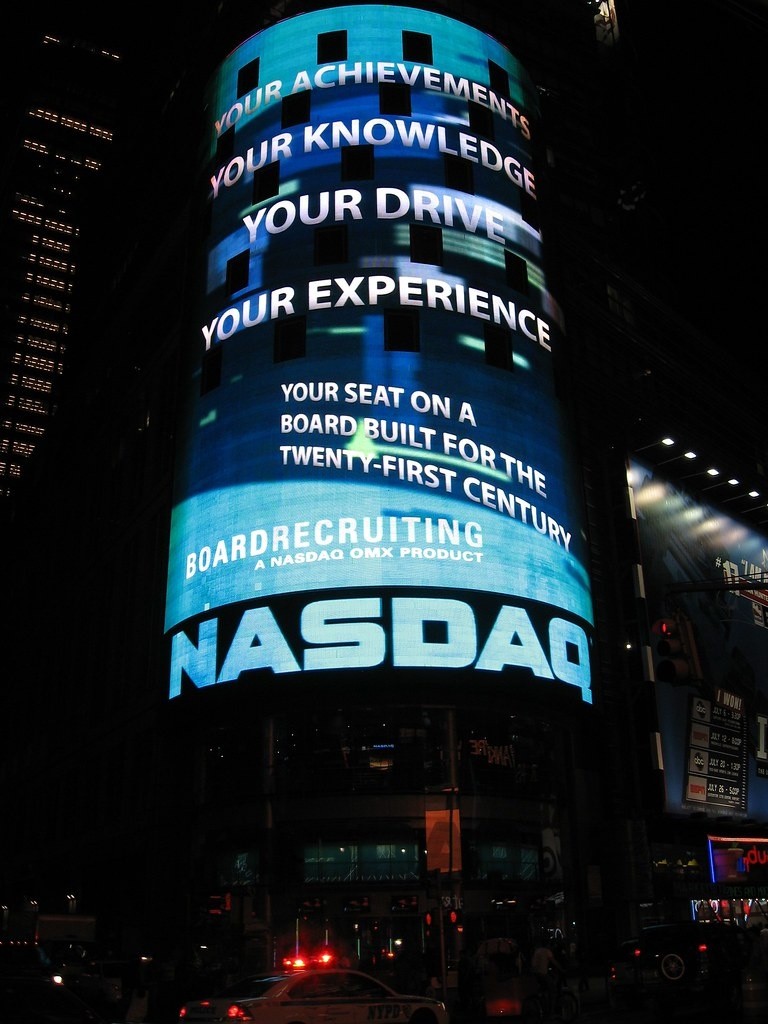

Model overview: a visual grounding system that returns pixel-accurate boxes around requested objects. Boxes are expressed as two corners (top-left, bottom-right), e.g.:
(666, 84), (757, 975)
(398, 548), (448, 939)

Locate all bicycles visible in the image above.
(523, 968), (579, 1024)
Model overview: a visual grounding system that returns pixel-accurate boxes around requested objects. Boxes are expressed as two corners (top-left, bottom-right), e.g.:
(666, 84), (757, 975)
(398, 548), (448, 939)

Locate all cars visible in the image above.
(176, 954), (450, 1024)
(607, 925), (740, 1002)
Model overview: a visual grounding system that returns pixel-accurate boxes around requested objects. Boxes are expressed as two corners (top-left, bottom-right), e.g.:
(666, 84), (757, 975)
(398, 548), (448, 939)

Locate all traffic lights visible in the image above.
(450, 912), (464, 934)
(425, 913), (432, 936)
